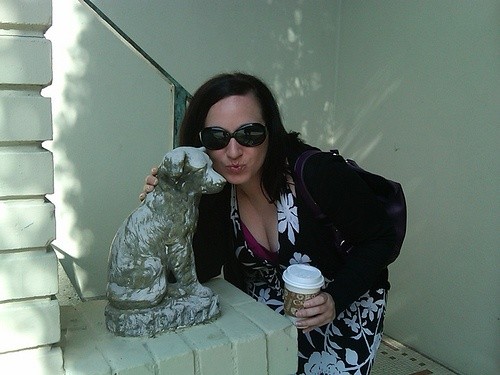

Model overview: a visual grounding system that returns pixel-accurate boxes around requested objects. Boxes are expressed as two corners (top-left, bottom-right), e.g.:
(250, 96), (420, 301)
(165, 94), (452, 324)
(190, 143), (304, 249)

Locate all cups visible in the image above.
(282, 263), (325, 329)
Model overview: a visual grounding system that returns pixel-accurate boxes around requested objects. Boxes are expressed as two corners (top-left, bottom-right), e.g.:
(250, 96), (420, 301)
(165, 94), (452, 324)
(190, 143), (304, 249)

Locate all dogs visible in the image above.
(104, 146), (226, 312)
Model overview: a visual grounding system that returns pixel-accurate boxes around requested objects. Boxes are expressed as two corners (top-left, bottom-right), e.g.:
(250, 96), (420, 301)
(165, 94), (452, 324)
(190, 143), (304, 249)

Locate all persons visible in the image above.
(140, 72), (407, 375)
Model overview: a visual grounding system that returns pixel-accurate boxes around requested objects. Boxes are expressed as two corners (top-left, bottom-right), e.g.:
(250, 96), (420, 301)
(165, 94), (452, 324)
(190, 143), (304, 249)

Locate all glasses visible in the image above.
(198, 123), (267, 150)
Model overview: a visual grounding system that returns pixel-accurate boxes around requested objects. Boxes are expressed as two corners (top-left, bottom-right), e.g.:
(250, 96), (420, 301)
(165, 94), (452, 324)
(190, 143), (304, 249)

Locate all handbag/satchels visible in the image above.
(295, 148), (408, 267)
